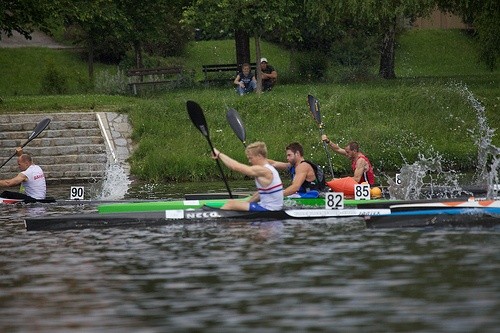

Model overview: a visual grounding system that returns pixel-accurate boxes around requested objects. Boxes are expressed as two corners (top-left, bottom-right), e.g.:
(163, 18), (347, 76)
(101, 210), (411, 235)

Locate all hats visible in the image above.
(261, 58), (267, 63)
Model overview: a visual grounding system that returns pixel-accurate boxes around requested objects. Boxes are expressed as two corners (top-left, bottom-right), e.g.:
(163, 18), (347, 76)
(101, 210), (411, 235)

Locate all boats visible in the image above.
(23, 198), (500, 231)
(0, 187), (500, 205)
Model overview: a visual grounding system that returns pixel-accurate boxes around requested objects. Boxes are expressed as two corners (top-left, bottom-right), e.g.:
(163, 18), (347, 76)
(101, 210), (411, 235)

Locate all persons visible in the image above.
(210, 140), (386, 210)
(0, 147), (47, 203)
(234, 57), (279, 97)
